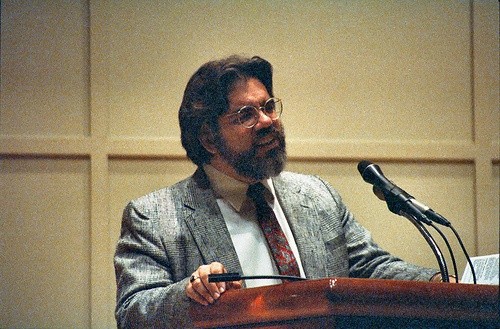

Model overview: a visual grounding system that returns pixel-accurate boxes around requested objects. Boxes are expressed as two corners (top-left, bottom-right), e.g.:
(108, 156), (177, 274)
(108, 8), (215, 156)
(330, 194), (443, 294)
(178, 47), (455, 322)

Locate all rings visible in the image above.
(189, 275), (200, 285)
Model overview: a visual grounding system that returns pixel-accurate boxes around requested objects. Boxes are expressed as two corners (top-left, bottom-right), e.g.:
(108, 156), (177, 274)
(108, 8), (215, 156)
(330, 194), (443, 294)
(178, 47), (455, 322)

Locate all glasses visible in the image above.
(224, 97), (283, 128)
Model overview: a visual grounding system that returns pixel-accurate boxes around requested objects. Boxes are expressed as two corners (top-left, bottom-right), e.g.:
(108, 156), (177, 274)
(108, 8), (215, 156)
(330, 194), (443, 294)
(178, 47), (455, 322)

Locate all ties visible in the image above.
(247, 182), (301, 283)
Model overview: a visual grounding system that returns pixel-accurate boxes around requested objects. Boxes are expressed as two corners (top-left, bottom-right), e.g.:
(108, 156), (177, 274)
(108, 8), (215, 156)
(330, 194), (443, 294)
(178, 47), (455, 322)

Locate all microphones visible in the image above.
(208, 272), (308, 283)
(358, 160), (476, 283)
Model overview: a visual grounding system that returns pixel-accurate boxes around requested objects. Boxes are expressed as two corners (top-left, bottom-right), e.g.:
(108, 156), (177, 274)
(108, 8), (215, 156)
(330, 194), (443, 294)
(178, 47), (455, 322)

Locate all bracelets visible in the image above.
(435, 274), (457, 283)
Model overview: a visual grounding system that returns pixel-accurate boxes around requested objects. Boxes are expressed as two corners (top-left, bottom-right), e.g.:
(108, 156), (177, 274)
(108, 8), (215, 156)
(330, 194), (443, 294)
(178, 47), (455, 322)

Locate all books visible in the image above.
(461, 254), (499, 285)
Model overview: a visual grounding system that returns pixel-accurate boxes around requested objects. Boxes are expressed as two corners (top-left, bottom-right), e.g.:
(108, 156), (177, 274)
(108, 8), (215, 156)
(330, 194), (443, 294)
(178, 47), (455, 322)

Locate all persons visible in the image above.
(113, 55), (461, 329)
